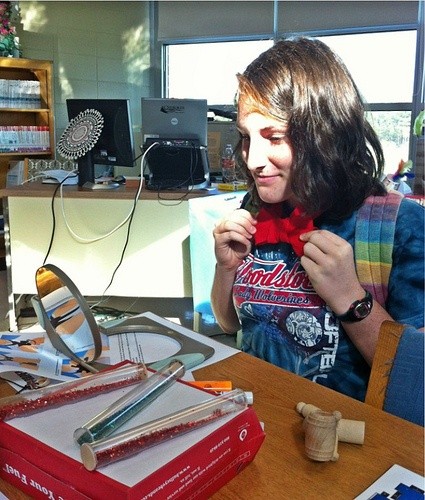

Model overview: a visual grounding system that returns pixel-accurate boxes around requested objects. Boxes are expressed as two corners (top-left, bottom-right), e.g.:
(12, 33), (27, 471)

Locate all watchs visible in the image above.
(333, 289), (373, 323)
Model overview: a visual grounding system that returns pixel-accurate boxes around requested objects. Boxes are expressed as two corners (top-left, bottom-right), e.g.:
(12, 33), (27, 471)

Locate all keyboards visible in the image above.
(102, 176), (145, 181)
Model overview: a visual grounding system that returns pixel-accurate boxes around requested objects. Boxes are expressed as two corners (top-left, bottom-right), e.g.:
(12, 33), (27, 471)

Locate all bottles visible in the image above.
(222, 144), (235, 182)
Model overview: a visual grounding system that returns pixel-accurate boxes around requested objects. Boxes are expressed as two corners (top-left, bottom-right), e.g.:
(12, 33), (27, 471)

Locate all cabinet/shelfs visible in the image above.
(0, 57), (55, 183)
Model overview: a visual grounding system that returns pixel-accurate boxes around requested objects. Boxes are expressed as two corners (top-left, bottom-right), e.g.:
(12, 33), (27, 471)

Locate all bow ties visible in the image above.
(253, 201), (319, 257)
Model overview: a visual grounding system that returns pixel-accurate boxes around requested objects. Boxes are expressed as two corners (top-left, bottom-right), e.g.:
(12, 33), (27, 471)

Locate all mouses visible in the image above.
(112, 175), (126, 184)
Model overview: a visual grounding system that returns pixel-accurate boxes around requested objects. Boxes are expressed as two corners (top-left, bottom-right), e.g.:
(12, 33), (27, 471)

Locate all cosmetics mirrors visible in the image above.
(30, 264), (215, 374)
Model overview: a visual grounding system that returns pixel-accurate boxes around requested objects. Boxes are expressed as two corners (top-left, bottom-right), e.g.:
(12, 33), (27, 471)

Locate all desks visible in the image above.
(0, 177), (246, 337)
(0, 311), (425, 500)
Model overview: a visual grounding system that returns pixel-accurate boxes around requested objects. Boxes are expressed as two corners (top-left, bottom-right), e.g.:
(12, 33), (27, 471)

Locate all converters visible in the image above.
(20, 307), (37, 317)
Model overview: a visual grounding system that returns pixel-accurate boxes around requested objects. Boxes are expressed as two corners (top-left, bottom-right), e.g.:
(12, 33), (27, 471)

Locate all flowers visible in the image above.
(0, 0), (23, 57)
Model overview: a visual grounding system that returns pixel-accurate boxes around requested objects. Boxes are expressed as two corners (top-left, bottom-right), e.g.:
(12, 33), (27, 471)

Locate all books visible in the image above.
(6, 160), (24, 188)
(0, 126), (51, 153)
(0, 79), (40, 109)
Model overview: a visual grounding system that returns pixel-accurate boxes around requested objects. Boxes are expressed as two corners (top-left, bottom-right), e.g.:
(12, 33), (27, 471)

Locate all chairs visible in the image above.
(364, 319), (425, 428)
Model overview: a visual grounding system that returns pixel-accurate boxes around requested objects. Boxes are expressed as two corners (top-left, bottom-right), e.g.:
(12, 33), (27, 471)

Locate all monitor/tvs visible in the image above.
(66, 98), (136, 187)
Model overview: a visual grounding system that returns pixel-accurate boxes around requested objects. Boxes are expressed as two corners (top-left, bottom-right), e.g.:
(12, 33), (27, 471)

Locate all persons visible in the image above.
(210, 37), (425, 403)
